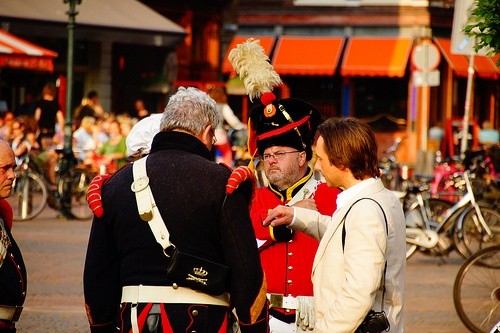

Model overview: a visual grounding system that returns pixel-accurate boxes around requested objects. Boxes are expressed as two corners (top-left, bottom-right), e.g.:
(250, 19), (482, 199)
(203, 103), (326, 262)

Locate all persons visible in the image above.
(0, 139), (29, 333)
(0, 100), (150, 193)
(249, 97), (345, 333)
(35, 83), (65, 149)
(262, 115), (407, 333)
(71, 89), (105, 130)
(83, 84), (270, 333)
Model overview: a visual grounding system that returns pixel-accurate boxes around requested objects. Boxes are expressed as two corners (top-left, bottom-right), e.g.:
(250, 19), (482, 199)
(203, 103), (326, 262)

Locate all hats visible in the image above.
(229, 38), (319, 160)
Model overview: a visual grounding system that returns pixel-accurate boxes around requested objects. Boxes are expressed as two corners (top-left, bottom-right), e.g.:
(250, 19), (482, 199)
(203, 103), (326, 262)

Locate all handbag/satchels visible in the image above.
(165, 248), (239, 298)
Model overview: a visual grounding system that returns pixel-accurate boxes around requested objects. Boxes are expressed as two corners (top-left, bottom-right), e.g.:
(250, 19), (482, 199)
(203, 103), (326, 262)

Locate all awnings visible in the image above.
(340, 35), (413, 78)
(0, 28), (59, 73)
(220, 35), (277, 78)
(433, 36), (500, 80)
(270, 35), (346, 76)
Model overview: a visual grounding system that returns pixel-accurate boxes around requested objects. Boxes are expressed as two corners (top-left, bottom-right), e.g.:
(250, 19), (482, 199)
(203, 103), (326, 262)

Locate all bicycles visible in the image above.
(378, 135), (500, 333)
(5, 139), (94, 220)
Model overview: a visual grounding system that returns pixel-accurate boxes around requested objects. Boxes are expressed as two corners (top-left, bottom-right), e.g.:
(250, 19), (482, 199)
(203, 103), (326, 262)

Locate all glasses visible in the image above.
(261, 149), (304, 160)
